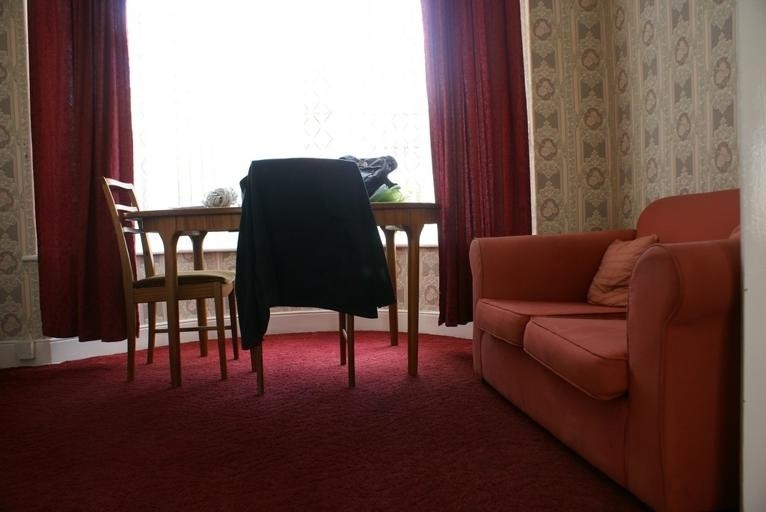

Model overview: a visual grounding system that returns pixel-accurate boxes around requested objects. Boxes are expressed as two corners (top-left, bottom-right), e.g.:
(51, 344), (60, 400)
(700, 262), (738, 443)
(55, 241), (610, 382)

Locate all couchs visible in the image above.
(469, 186), (741, 512)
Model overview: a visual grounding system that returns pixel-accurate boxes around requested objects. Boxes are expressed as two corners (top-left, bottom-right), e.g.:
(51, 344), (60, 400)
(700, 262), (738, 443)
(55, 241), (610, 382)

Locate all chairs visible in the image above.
(250, 159), (357, 395)
(99, 175), (239, 379)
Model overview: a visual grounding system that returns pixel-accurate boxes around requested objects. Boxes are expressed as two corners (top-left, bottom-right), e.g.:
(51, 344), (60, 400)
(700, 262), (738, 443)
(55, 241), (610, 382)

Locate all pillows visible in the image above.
(588, 234), (658, 304)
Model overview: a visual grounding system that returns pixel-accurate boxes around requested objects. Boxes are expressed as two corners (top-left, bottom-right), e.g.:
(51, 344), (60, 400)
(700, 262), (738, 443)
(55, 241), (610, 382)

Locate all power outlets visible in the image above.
(16, 340), (35, 360)
